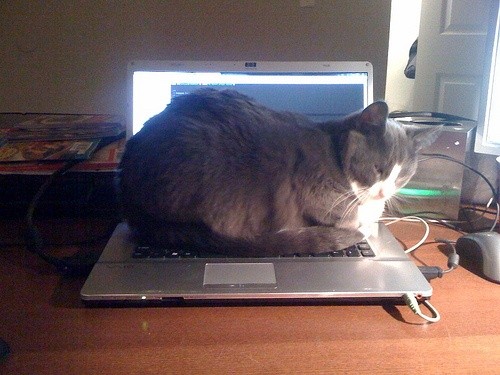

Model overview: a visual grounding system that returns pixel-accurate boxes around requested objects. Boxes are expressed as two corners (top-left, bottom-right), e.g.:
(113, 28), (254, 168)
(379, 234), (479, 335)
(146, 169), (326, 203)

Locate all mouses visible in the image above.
(456, 232), (500, 286)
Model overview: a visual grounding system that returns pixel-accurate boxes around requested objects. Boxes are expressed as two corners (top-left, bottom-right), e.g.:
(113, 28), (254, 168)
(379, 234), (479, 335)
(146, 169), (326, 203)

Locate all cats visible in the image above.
(118, 89), (444, 255)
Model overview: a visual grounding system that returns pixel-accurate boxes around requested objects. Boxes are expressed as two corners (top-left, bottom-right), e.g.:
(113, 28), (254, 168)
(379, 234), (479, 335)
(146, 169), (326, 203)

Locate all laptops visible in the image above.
(80, 61), (432, 308)
